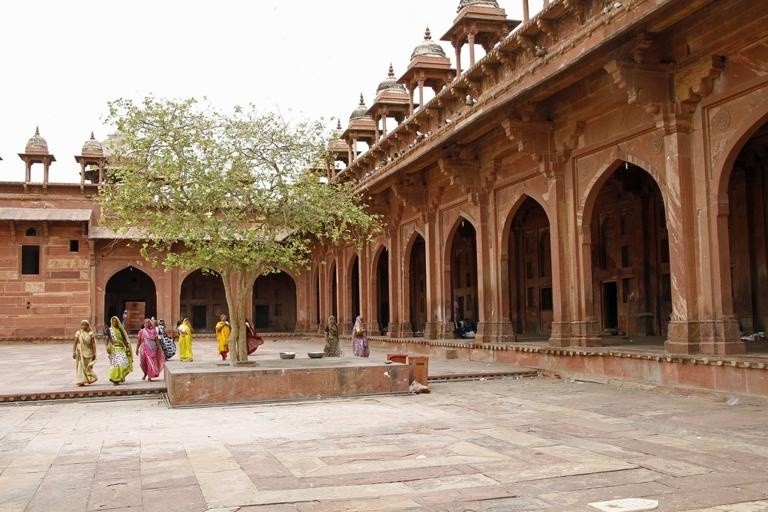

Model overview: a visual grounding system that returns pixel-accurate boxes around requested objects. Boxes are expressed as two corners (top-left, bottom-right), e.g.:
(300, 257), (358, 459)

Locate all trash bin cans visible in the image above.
(387, 353), (429, 386)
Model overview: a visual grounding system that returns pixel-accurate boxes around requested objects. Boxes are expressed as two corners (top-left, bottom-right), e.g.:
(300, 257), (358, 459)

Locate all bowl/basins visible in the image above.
(308, 352), (324, 358)
(279, 352), (296, 360)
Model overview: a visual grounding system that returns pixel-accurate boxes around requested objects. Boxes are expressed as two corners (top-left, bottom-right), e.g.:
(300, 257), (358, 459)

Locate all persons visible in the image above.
(214, 313), (232, 361)
(121, 310), (130, 334)
(352, 316), (371, 358)
(148, 315), (177, 361)
(244, 317), (263, 355)
(323, 314), (343, 356)
(135, 317), (166, 382)
(103, 316), (134, 385)
(72, 319), (98, 386)
(176, 316), (194, 363)
